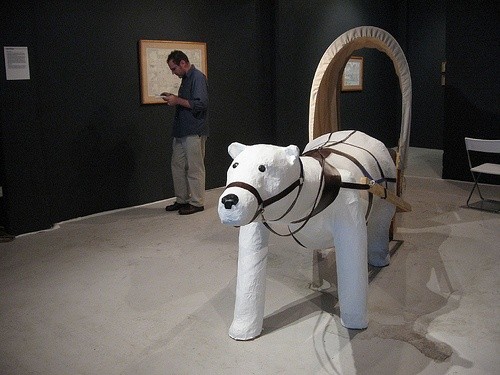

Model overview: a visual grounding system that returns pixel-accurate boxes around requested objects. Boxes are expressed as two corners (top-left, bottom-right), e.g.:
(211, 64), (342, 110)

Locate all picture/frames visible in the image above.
(139, 39), (207, 104)
(341, 56), (363, 91)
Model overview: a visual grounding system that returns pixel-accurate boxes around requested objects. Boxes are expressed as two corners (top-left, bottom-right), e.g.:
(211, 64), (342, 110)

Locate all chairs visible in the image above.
(464, 137), (500, 207)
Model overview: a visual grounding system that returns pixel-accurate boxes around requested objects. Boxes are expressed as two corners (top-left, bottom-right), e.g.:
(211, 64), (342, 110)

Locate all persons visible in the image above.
(160, 50), (211, 216)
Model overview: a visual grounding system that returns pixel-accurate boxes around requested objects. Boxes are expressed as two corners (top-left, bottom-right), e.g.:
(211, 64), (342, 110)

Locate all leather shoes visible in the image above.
(164, 201), (205, 215)
